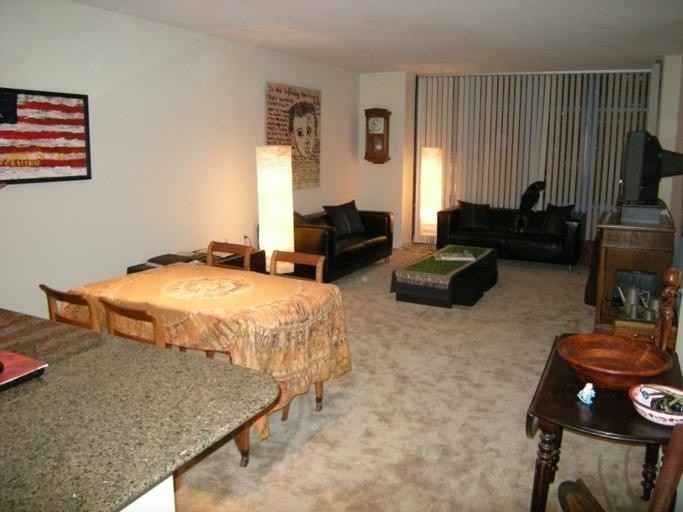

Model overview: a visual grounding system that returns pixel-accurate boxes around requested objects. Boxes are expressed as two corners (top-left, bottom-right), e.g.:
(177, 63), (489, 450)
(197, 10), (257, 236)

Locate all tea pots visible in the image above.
(640, 296), (663, 319)
(616, 285), (651, 317)
(638, 306), (657, 323)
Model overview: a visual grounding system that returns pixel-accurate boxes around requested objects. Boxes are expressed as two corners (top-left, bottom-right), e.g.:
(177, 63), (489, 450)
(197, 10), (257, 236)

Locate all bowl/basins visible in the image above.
(555, 333), (674, 391)
(627, 382), (683, 427)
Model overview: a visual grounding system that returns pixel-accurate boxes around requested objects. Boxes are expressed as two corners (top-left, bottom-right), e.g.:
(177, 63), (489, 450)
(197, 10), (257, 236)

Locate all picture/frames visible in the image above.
(0, 87), (91, 185)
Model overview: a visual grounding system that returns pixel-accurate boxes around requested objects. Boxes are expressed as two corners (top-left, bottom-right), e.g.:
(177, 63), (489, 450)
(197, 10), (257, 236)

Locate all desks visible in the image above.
(0, 307), (282, 512)
(60, 261), (352, 442)
(526, 333), (683, 512)
(127, 245), (265, 275)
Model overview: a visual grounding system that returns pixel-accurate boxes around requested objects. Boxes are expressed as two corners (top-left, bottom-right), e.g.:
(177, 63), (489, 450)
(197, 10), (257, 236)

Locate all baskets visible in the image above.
(612, 305), (678, 349)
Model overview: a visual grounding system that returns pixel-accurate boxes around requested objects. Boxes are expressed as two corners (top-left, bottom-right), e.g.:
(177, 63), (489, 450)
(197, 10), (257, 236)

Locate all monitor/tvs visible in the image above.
(616, 130), (683, 207)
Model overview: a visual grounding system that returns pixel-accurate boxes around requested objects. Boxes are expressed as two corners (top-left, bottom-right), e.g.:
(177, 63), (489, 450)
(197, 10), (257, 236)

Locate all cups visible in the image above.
(630, 304), (638, 320)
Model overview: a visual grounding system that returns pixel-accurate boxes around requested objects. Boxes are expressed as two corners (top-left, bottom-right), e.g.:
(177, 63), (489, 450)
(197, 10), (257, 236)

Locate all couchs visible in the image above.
(437, 205), (586, 272)
(294, 211), (393, 283)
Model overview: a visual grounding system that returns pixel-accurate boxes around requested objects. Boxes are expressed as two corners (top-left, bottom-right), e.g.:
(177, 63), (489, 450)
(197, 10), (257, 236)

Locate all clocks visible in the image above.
(363, 108), (392, 164)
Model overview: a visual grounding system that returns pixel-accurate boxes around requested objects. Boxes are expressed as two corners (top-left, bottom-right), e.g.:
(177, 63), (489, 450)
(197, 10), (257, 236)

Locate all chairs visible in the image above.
(39, 285), (101, 332)
(98, 296), (249, 491)
(207, 241), (251, 270)
(269, 251), (325, 421)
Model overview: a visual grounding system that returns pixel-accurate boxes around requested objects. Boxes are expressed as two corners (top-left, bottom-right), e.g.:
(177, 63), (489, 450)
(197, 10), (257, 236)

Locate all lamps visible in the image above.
(256, 145), (295, 274)
(419, 148), (445, 249)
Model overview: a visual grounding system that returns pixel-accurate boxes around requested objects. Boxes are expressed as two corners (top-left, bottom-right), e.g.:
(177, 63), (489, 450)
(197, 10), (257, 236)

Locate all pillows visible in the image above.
(457, 200), (494, 232)
(538, 202), (575, 240)
(322, 200), (368, 238)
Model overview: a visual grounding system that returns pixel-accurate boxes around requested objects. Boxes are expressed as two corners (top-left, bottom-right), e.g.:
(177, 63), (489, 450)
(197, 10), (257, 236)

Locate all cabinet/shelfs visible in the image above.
(612, 318), (677, 350)
(595, 207), (676, 323)
